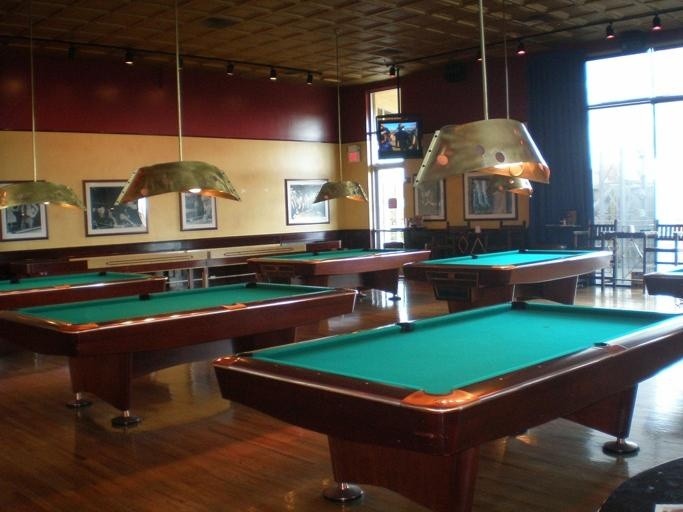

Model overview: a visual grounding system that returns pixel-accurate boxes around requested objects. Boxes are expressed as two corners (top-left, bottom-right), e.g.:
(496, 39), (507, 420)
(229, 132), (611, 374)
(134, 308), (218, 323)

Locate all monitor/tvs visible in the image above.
(375, 120), (423, 159)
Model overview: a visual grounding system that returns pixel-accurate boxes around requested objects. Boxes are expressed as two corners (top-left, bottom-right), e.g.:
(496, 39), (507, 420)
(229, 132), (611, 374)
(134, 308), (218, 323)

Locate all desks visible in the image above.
(601, 231), (657, 290)
(455, 233), (489, 256)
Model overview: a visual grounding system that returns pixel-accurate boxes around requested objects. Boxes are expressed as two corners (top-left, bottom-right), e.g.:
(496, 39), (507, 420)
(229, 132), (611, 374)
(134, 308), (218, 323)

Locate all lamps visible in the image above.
(390, 66), (396, 77)
(226, 63), (234, 76)
(652, 15), (662, 31)
(517, 42), (526, 55)
(125, 51), (133, 65)
(114, 0), (243, 206)
(485, 41), (534, 197)
(606, 25), (616, 39)
(269, 69), (277, 81)
(478, 50), (482, 61)
(0, 17), (87, 212)
(314, 28), (368, 204)
(179, 58), (183, 71)
(413, 0), (551, 187)
(69, 46), (76, 60)
(306, 75), (312, 85)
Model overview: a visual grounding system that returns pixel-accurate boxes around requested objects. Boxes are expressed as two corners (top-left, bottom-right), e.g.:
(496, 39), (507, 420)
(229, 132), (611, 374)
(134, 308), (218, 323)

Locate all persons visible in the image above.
(379, 124), (416, 154)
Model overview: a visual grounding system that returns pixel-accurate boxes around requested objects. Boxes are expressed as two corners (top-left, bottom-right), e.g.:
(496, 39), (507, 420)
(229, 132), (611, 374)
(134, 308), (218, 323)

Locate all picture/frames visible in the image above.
(0, 179), (48, 243)
(179, 192), (218, 231)
(462, 170), (519, 222)
(82, 178), (149, 237)
(285, 179), (330, 226)
(413, 174), (447, 223)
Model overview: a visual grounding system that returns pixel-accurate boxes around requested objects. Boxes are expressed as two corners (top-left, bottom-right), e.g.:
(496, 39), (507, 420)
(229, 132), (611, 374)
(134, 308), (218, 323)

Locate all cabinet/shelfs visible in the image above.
(545, 225), (580, 249)
(404, 227), (428, 281)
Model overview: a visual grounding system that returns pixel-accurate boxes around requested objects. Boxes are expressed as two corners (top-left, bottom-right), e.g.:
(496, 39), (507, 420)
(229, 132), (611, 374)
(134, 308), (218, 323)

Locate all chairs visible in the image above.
(643, 220), (683, 294)
(573, 220), (617, 290)
(432, 221), (470, 259)
(488, 220), (526, 252)
